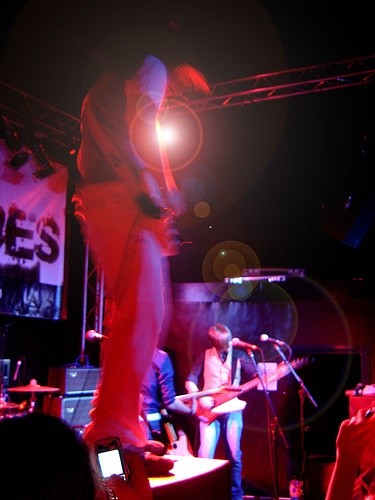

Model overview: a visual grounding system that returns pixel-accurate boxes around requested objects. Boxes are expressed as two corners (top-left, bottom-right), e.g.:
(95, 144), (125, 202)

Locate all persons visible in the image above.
(141, 348), (197, 454)
(76, 64), (209, 474)
(185, 323), (293, 500)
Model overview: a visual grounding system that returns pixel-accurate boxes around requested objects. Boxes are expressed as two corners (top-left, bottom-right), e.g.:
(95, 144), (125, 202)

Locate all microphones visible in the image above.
(259, 334), (289, 347)
(85, 330), (102, 343)
(13, 361), (22, 381)
(231, 337), (258, 350)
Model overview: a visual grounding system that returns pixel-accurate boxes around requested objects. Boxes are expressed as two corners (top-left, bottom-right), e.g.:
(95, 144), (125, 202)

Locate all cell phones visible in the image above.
(94, 437), (129, 500)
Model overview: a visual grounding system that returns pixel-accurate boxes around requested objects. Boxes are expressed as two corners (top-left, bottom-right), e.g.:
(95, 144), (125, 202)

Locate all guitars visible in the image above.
(139, 172), (186, 258)
(183, 356), (310, 427)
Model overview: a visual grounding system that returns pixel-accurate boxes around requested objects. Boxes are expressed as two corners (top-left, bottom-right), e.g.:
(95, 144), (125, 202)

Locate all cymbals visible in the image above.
(6, 384), (60, 392)
(0, 401), (30, 409)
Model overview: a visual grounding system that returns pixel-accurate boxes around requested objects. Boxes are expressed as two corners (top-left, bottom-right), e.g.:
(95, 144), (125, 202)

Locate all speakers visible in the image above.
(287, 346), (362, 464)
(44, 395), (94, 437)
(48, 368), (99, 394)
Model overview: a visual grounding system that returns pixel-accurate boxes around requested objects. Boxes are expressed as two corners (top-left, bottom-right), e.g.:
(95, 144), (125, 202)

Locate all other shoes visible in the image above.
(142, 452), (173, 475)
(144, 438), (167, 456)
